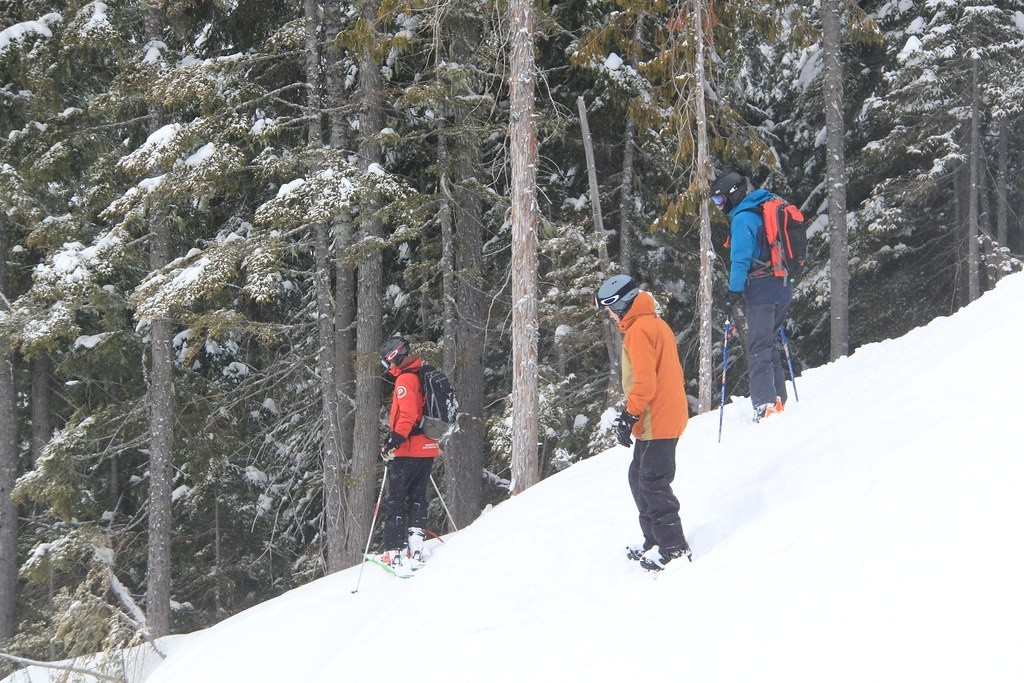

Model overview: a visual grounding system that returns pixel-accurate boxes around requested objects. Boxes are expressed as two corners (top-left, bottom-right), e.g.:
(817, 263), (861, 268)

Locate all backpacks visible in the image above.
(739, 195), (808, 278)
(399, 365), (460, 441)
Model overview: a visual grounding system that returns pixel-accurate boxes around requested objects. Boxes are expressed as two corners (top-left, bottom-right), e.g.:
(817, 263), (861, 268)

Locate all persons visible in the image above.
(379, 336), (439, 567)
(594, 275), (693, 572)
(710, 171), (793, 423)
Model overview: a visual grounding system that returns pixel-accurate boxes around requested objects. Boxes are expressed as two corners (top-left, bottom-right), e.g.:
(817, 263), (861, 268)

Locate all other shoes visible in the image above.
(407, 549), (420, 562)
(752, 404), (773, 423)
(640, 543), (691, 570)
(626, 547), (649, 561)
(377, 550), (400, 567)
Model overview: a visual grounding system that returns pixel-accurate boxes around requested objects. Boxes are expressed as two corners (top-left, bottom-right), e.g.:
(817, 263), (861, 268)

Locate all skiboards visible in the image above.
(363, 549), (428, 579)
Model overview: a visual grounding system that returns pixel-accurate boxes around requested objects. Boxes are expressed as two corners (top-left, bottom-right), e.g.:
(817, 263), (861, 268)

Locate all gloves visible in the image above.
(716, 291), (742, 315)
(382, 438), (396, 462)
(615, 409), (640, 447)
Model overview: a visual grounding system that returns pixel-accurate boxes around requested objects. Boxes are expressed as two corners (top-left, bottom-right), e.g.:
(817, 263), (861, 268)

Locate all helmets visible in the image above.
(599, 273), (639, 315)
(379, 337), (408, 371)
(710, 172), (748, 214)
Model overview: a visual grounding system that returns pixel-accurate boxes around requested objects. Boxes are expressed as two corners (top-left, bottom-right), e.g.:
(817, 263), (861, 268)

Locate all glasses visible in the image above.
(593, 296), (600, 311)
(380, 359), (390, 371)
(711, 193), (727, 208)
